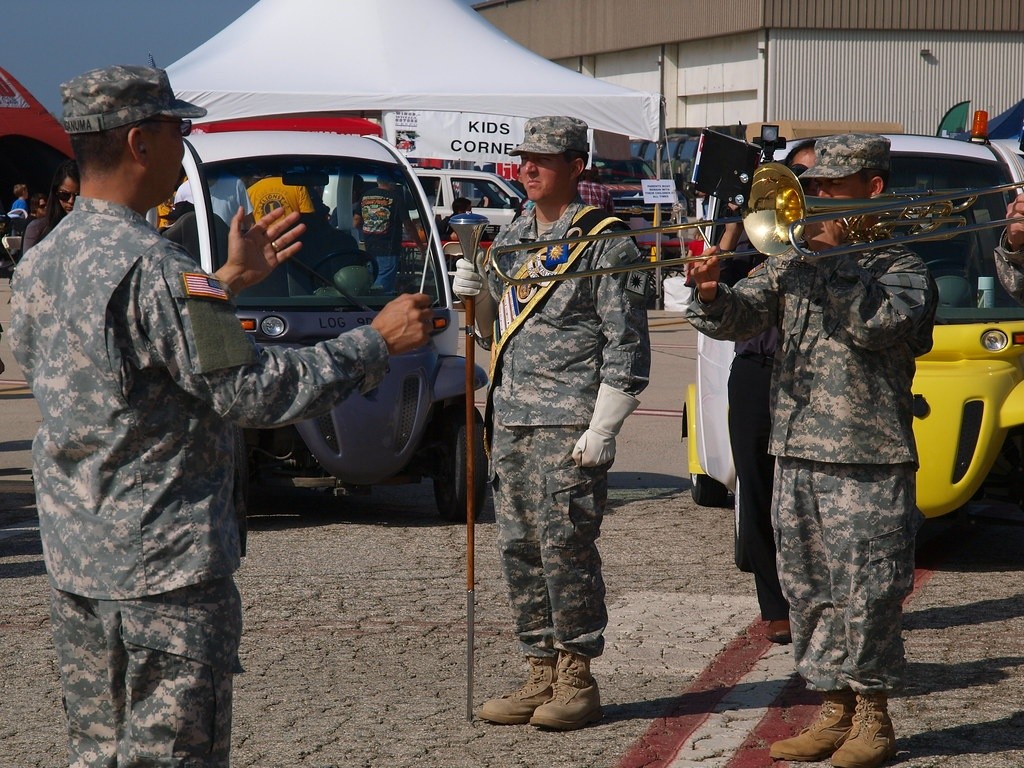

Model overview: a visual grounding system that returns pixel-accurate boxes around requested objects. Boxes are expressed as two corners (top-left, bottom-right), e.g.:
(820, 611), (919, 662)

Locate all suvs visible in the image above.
(585, 152), (673, 220)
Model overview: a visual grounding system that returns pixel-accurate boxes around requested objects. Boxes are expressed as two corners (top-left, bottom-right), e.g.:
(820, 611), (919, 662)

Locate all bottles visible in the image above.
(976, 277), (995, 309)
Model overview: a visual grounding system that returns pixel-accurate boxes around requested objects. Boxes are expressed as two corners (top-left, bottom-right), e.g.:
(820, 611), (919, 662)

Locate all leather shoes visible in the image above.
(765, 620), (792, 641)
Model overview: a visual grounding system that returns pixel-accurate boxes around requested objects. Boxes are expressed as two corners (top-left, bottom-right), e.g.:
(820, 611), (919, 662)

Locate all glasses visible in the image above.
(147, 119), (192, 136)
(57, 190), (79, 202)
(792, 164), (811, 187)
(38, 204), (46, 210)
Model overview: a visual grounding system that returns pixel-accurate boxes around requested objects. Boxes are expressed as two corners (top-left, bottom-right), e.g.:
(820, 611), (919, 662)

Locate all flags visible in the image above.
(935, 101), (970, 137)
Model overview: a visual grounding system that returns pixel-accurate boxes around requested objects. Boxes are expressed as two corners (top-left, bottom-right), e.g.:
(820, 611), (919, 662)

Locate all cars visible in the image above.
(681, 134), (1023, 549)
(144, 117), (494, 524)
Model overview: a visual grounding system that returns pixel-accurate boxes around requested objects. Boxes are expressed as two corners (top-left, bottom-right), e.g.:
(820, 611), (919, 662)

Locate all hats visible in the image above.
(509, 117), (590, 157)
(796, 133), (892, 181)
(61, 64), (207, 133)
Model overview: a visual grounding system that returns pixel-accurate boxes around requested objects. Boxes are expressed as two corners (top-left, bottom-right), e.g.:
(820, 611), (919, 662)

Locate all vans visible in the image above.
(322, 167), (528, 244)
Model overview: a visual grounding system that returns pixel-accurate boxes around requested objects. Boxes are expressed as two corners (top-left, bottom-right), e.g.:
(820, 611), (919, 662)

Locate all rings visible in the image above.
(271, 242), (279, 252)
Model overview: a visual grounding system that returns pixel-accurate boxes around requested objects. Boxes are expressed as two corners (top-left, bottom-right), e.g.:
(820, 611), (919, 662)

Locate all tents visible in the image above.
(163, 0), (663, 310)
(0, 66), (77, 161)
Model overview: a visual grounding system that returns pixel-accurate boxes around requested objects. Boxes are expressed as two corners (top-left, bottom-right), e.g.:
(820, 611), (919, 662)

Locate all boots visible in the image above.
(832, 695), (897, 767)
(769, 689), (859, 761)
(530, 652), (603, 728)
(480, 658), (558, 724)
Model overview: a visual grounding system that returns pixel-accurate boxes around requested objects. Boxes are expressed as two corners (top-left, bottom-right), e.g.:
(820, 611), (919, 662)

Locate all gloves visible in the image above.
(453, 252), (497, 338)
(572, 384), (641, 468)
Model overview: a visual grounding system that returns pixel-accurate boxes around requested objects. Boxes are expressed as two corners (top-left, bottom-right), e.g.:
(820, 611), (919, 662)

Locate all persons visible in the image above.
(329, 174), (365, 244)
(452, 115), (652, 732)
(23, 159), (80, 256)
(174, 176), (255, 231)
(994, 191), (1024, 312)
(352, 173), (425, 295)
(682, 130), (939, 768)
(6, 66), (436, 768)
(578, 164), (614, 209)
(247, 171), (314, 227)
(21, 193), (47, 258)
(11, 184), (28, 214)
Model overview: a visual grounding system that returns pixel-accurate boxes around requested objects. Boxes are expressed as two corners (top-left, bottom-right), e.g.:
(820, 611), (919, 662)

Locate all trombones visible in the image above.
(490, 160), (978, 287)
(788, 178), (1024, 263)
